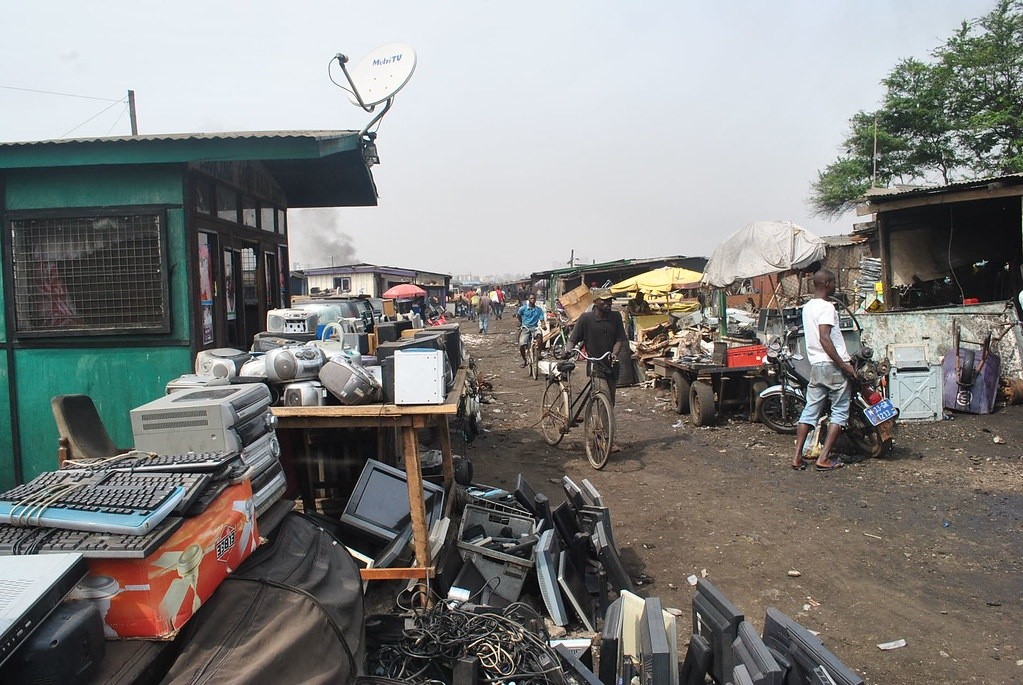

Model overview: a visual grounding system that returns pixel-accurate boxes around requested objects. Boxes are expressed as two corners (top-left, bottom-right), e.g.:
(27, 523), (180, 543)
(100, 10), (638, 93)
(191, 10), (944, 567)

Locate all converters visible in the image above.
(453, 655), (480, 685)
(539, 655), (563, 685)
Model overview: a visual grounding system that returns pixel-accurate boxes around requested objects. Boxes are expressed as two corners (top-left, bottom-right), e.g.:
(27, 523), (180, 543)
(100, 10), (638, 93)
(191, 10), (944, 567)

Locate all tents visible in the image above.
(701, 219), (826, 288)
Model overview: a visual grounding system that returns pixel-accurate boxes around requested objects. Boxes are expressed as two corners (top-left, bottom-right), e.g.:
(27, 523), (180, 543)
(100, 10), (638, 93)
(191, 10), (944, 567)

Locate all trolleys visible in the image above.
(940, 316), (1003, 414)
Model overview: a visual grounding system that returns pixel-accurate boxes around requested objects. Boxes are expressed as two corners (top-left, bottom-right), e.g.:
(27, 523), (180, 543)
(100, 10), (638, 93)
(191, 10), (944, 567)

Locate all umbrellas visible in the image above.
(607, 266), (704, 314)
(382, 283), (426, 299)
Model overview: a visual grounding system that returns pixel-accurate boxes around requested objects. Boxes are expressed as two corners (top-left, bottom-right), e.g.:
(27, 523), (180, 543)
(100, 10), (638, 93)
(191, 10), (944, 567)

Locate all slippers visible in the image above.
(816, 460), (842, 470)
(794, 461), (807, 470)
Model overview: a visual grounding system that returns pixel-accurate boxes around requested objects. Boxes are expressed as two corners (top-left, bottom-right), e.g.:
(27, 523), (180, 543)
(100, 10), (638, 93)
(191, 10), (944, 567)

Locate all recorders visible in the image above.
(195, 307), (384, 406)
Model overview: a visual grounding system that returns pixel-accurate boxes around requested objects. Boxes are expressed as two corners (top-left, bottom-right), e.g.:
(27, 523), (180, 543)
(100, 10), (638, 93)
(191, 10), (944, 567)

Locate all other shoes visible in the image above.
(538, 355), (544, 359)
(594, 436), (620, 453)
(520, 359), (527, 368)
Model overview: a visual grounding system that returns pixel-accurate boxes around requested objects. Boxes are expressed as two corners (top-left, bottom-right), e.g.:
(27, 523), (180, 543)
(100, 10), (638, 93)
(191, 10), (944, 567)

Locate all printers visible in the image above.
(131, 384), (296, 538)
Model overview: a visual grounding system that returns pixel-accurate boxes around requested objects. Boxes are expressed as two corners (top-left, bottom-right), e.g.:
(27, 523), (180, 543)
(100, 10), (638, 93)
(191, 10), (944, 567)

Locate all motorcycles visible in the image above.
(757, 323), (901, 458)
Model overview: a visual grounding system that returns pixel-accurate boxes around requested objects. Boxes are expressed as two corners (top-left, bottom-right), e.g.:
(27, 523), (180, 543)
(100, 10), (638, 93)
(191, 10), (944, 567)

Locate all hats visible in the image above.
(593, 288), (617, 301)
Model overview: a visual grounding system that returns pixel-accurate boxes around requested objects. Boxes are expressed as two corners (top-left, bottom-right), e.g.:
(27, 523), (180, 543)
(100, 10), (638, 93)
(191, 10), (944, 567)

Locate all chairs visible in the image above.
(51, 394), (135, 469)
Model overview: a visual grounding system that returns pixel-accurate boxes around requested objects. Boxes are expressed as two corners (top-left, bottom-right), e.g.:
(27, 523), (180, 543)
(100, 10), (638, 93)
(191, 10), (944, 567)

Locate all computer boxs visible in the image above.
(393, 348), (447, 405)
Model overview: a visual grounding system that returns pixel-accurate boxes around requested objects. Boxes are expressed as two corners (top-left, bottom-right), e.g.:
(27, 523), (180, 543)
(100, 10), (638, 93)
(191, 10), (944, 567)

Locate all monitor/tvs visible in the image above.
(376, 322), (461, 404)
(341, 457), (865, 685)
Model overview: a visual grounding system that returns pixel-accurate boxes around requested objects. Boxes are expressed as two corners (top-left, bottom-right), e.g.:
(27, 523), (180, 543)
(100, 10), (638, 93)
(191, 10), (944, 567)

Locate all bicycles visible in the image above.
(517, 325), (545, 381)
(531, 349), (615, 471)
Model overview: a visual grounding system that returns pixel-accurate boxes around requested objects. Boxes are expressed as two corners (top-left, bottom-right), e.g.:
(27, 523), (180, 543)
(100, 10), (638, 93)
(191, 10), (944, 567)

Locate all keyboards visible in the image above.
(0, 449), (252, 558)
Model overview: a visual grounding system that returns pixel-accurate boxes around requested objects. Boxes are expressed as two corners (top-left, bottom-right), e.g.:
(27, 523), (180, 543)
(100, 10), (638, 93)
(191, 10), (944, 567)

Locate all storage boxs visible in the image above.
(860, 282), (883, 313)
(632, 315), (669, 341)
(726, 345), (767, 367)
(68, 479), (268, 639)
(458, 506), (536, 604)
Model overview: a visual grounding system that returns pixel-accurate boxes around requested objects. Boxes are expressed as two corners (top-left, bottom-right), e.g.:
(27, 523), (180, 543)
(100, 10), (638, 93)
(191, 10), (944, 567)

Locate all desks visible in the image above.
(271, 365), (469, 608)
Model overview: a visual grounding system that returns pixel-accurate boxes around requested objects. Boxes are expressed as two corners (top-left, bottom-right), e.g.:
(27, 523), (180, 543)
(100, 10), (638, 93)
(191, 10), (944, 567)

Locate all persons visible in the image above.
(561, 288), (628, 452)
(792, 271), (859, 471)
(628, 291), (650, 341)
(465, 286), (507, 335)
(516, 293), (547, 368)
